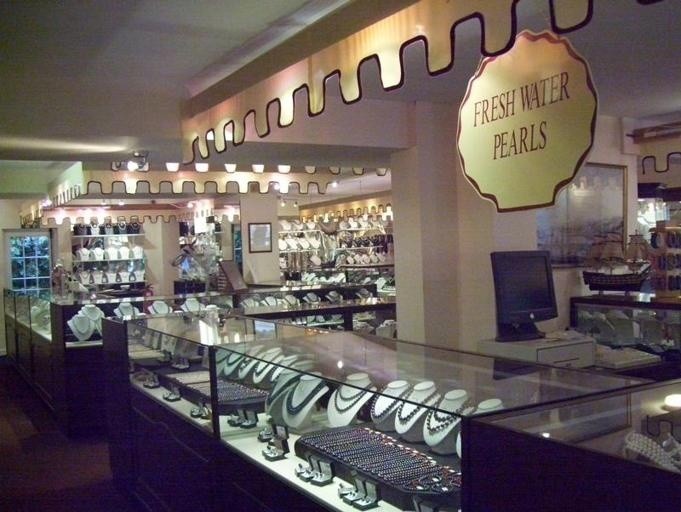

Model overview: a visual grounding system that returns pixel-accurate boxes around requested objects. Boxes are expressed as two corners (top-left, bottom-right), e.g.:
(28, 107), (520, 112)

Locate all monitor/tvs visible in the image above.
(489, 249), (560, 342)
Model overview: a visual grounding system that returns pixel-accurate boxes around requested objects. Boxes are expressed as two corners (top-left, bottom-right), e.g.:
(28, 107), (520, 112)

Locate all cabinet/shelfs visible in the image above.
(0, 213), (681, 511)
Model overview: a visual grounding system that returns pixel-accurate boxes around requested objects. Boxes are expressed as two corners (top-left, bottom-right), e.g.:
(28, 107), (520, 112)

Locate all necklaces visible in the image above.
(73, 221), (147, 286)
(211, 342), (484, 494)
(625, 430), (681, 474)
(66, 286), (377, 341)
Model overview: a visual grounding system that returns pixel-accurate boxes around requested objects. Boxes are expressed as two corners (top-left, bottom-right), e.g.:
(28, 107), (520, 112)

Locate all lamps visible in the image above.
(128, 151), (149, 172)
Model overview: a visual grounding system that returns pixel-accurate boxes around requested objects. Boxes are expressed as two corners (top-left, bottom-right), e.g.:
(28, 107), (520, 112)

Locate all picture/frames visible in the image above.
(538, 148), (626, 264)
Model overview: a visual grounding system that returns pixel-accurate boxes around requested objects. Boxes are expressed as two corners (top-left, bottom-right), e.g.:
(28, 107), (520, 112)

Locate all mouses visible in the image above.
(635, 342), (666, 355)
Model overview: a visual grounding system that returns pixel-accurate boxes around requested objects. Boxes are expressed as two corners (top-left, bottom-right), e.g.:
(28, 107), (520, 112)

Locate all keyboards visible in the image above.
(594, 346), (662, 369)
